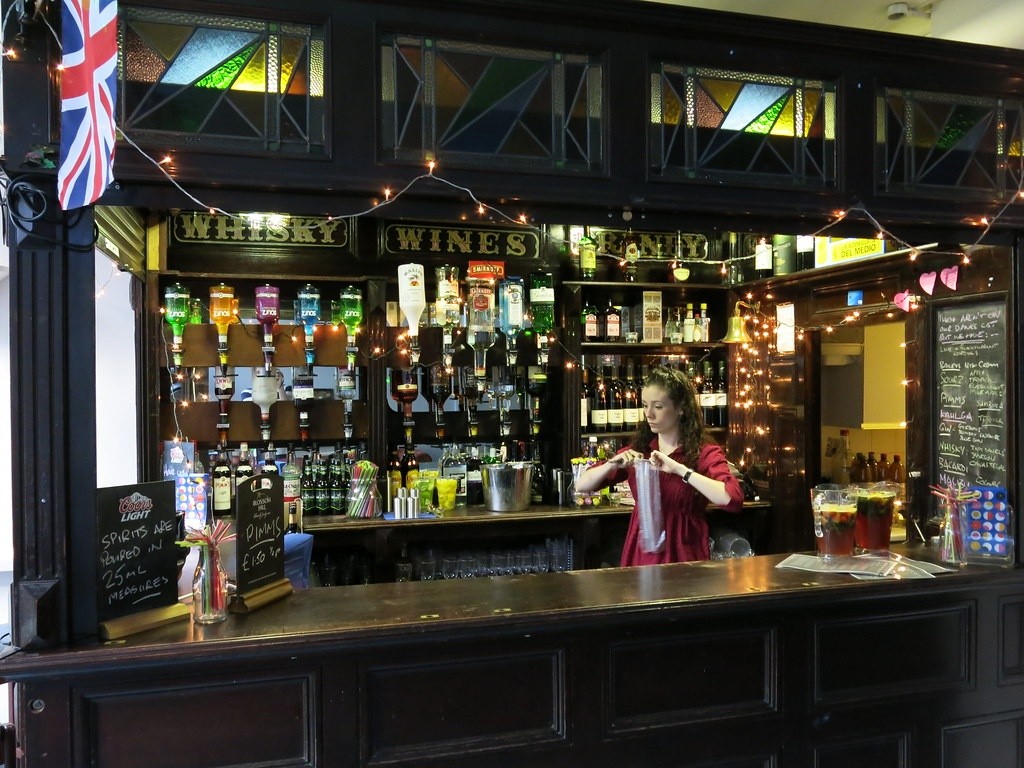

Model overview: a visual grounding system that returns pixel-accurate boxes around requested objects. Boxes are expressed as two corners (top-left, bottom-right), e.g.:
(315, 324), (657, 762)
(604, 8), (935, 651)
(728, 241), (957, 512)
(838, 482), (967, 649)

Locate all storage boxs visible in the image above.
(643, 290), (662, 343)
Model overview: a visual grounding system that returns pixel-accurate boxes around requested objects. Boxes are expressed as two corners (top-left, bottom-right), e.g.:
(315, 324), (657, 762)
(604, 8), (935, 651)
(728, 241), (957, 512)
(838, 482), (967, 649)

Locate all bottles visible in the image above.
(163, 283), (364, 440)
(183, 440), (383, 518)
(941, 501), (968, 567)
(440, 440), (566, 507)
(756, 232), (797, 280)
(190, 544), (228, 626)
(386, 262), (556, 441)
(386, 442), (418, 519)
(307, 543), (414, 587)
(831, 430), (907, 486)
(578, 226), (743, 463)
(282, 502), (302, 534)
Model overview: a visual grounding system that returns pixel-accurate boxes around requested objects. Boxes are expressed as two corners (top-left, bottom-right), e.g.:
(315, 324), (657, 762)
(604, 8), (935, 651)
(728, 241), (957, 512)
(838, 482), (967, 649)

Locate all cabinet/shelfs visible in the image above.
(146, 274), (773, 584)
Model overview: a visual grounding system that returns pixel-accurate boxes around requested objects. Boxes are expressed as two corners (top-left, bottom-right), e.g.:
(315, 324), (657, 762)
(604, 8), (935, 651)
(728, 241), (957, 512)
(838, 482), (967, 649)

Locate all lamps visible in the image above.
(721, 300), (759, 343)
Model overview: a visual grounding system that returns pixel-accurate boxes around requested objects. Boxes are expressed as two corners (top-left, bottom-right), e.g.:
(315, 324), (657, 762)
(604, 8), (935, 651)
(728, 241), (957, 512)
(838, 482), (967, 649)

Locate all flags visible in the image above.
(56, 0), (117, 210)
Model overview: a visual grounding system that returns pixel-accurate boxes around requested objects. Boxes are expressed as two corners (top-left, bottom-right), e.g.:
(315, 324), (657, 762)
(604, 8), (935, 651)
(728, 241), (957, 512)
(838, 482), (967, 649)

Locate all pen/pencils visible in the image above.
(347, 461), (379, 520)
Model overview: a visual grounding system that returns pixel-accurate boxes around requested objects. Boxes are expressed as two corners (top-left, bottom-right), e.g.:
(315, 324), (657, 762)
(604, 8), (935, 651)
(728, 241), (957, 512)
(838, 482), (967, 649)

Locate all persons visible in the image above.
(574, 363), (745, 566)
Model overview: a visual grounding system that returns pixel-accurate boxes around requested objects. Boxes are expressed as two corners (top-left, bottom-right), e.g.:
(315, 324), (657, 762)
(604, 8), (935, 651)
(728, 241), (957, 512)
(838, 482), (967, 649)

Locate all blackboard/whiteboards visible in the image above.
(930, 291), (1015, 527)
(226, 473), (293, 616)
(97, 479), (191, 640)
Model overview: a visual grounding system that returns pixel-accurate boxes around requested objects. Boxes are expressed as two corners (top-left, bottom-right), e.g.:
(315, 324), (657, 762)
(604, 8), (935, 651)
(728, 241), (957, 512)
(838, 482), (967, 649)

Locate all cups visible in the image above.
(810, 484), (896, 560)
(669, 332), (683, 345)
(607, 494), (622, 508)
(415, 477), (457, 512)
(709, 528), (752, 560)
(418, 537), (576, 578)
(627, 331), (638, 344)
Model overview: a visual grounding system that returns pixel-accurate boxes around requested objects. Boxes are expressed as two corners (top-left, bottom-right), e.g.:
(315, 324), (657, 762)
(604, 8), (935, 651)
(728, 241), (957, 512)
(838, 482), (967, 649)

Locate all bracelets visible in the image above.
(682, 469), (695, 483)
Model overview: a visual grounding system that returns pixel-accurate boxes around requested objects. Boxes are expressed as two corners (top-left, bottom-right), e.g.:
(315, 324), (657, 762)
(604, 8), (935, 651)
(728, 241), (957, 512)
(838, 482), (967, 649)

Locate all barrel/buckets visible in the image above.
(480, 463), (535, 511)
